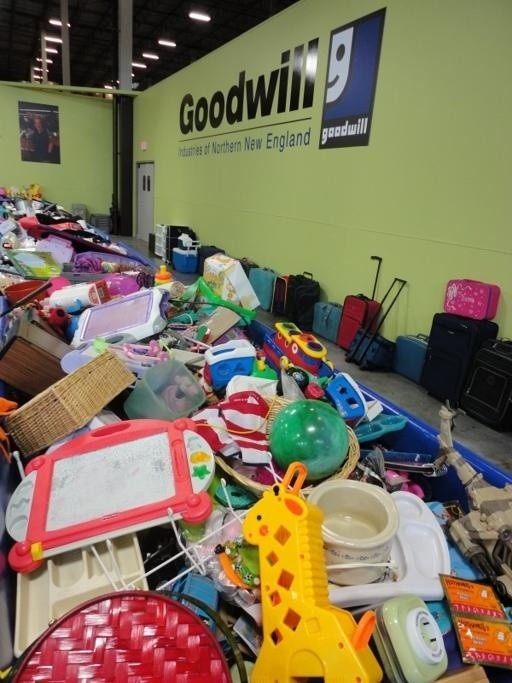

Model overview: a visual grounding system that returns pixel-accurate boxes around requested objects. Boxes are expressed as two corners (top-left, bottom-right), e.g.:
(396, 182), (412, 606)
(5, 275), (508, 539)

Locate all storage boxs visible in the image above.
(171, 247), (197, 274)
(122, 358), (207, 423)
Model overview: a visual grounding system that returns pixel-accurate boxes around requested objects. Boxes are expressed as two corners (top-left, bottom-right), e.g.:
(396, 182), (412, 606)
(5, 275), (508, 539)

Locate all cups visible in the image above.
(306, 478), (400, 588)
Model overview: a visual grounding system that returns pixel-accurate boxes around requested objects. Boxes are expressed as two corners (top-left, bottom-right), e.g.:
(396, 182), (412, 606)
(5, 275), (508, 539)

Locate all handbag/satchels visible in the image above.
(311, 300), (344, 343)
(445, 278), (500, 322)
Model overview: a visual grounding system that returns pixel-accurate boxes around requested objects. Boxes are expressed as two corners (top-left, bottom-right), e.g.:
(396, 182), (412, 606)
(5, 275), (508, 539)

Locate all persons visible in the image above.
(22, 115), (56, 161)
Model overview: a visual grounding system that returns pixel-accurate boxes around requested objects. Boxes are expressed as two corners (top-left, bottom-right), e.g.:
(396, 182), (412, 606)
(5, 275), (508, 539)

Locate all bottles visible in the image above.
(154, 265), (172, 286)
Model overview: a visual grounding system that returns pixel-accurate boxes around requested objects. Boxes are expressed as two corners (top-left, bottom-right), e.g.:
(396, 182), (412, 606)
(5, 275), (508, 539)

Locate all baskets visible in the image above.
(202, 394), (361, 496)
(2, 349), (139, 456)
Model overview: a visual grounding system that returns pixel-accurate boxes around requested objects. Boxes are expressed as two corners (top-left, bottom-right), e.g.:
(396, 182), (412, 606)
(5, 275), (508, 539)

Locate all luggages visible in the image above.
(338, 256), (382, 349)
(458, 339), (512, 432)
(421, 311), (499, 408)
(287, 270), (320, 331)
(345, 277), (409, 372)
(247, 265), (277, 312)
(198, 244), (225, 278)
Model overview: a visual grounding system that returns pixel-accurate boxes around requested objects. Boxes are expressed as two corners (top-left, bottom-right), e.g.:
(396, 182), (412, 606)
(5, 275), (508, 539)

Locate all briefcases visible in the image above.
(392, 330), (431, 386)
